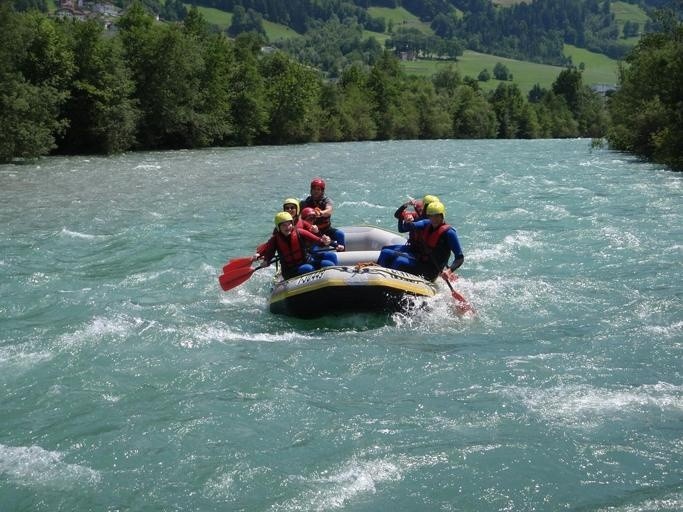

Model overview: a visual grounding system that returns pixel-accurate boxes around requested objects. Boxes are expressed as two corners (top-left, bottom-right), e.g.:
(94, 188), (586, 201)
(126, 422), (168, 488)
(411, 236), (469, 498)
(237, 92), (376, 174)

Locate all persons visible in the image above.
(256, 178), (345, 281)
(374, 193), (463, 285)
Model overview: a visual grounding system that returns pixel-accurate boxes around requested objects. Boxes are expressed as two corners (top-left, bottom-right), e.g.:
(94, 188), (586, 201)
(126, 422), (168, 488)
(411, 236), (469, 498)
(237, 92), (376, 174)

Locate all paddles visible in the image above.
(219, 238), (323, 292)
(400, 210), (476, 316)
(411, 203), (461, 281)
(222, 254), (263, 277)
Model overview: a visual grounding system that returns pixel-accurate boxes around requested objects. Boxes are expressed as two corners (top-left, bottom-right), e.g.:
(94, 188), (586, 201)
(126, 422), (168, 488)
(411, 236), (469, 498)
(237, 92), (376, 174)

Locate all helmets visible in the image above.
(274, 197), (316, 233)
(414, 195), (446, 222)
(311, 179), (325, 191)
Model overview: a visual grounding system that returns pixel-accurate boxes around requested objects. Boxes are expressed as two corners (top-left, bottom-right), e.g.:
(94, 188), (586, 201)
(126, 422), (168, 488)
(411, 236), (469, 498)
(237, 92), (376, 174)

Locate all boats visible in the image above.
(269, 223), (440, 320)
